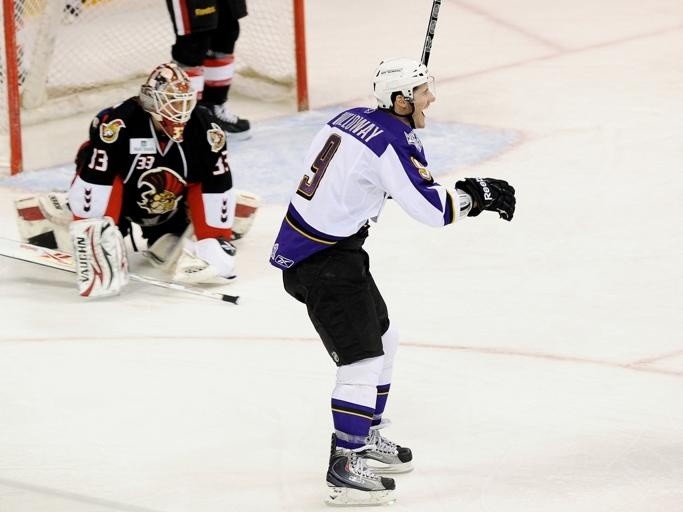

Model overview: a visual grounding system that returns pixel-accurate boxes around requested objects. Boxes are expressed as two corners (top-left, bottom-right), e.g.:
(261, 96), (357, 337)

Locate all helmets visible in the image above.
(373, 55), (432, 110)
(139, 61), (196, 122)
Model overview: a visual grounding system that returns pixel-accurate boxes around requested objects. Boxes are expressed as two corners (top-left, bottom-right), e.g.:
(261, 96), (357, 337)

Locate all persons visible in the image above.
(17, 62), (248, 296)
(166, 0), (251, 141)
(269, 58), (516, 492)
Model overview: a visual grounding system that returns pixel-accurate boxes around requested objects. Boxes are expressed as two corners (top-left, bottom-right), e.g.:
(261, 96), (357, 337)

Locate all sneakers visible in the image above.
(207, 105), (251, 134)
(358, 428), (413, 466)
(322, 449), (396, 492)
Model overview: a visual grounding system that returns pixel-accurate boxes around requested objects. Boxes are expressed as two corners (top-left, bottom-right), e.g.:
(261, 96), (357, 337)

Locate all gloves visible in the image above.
(455, 176), (518, 222)
